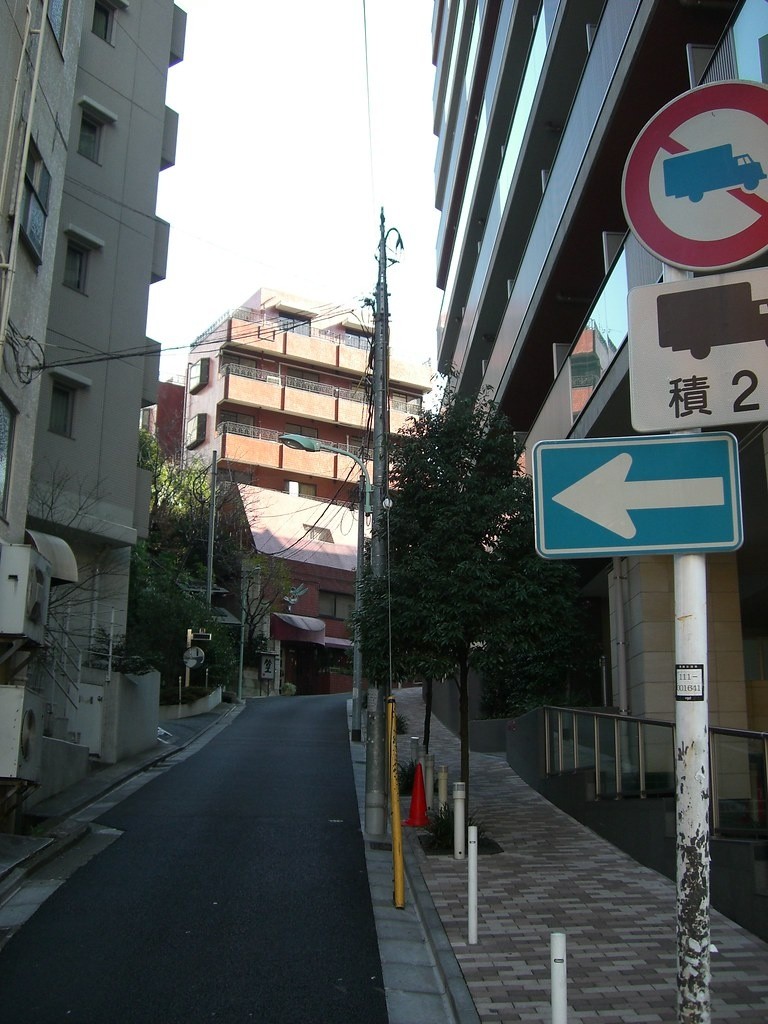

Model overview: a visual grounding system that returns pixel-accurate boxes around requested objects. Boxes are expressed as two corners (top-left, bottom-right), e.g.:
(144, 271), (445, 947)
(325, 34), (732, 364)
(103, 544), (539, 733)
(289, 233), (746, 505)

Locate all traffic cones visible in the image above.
(400, 762), (432, 827)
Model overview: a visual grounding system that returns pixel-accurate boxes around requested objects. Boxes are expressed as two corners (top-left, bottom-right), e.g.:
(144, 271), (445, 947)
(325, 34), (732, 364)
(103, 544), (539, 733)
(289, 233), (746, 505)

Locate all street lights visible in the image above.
(238, 567), (261, 699)
(278, 433), (387, 835)
